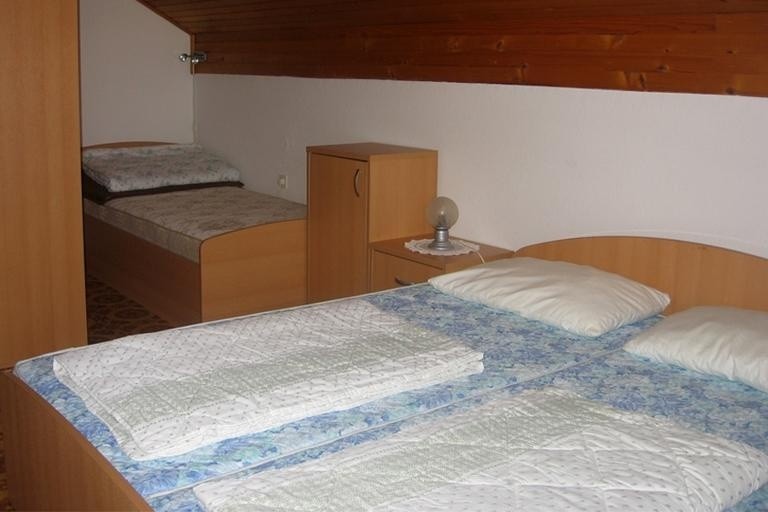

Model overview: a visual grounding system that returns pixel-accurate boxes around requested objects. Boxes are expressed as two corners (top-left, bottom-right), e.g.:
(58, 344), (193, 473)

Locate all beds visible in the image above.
(77, 141), (310, 329)
(0, 231), (768, 512)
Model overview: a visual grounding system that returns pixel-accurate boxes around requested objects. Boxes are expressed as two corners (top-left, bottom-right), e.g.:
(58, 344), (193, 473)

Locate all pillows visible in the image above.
(426, 252), (674, 341)
(621, 296), (768, 395)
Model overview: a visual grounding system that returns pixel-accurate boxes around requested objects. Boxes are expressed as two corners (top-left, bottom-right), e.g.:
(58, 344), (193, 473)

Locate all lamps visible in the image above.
(417, 193), (465, 254)
(174, 50), (211, 64)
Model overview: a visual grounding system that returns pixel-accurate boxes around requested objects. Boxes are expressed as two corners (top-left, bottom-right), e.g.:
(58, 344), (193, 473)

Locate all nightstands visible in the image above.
(358, 226), (517, 295)
(299, 140), (439, 308)
